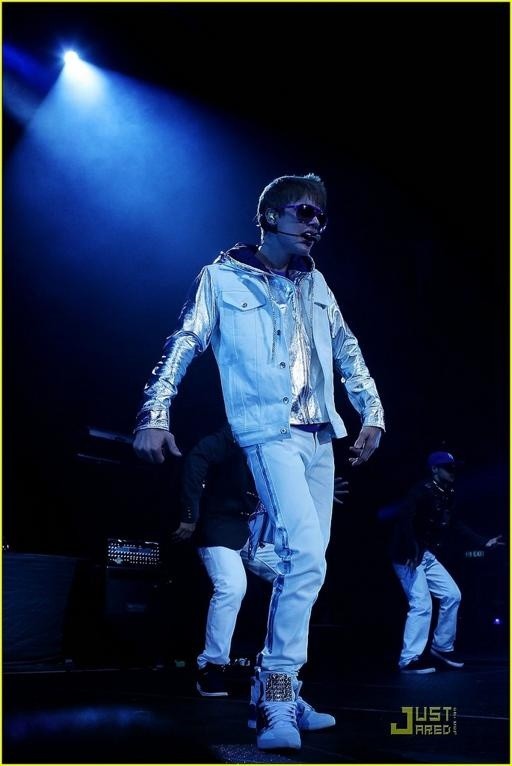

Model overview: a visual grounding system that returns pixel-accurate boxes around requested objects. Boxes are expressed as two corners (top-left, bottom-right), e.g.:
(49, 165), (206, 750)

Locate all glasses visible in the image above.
(272, 204), (328, 229)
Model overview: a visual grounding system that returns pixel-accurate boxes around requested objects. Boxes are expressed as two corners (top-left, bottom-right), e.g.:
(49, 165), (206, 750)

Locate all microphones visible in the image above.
(258, 215), (322, 242)
(85, 426), (170, 458)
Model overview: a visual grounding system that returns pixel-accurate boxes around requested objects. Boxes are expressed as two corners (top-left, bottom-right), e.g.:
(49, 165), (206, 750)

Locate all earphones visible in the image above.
(267, 213), (276, 221)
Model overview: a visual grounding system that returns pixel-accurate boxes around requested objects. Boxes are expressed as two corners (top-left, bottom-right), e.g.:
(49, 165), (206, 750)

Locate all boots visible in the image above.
(248, 671), (336, 749)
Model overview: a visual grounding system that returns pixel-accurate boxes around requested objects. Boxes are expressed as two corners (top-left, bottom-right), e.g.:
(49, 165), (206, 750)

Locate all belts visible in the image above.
(291, 424), (328, 432)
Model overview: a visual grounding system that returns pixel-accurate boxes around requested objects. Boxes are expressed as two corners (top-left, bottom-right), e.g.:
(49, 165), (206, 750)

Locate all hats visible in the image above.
(426, 452), (464, 469)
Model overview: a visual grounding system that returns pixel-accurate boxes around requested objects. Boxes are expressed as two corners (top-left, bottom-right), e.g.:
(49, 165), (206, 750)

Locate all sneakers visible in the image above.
(196, 661), (229, 697)
(401, 647), (464, 675)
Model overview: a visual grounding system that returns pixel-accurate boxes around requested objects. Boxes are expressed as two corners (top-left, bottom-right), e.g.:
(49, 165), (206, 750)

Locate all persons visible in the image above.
(172, 421), (285, 699)
(376, 448), (504, 675)
(125, 171), (389, 750)
